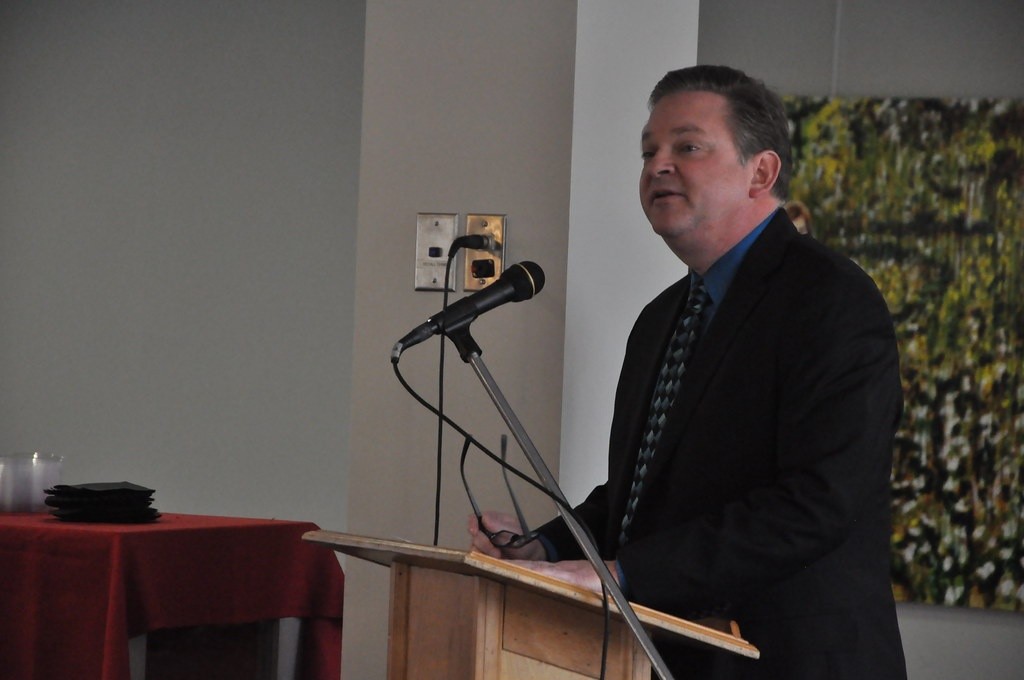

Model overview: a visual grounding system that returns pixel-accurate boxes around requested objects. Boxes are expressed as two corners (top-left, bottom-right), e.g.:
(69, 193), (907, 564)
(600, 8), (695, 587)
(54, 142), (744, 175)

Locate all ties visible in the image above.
(617, 282), (707, 543)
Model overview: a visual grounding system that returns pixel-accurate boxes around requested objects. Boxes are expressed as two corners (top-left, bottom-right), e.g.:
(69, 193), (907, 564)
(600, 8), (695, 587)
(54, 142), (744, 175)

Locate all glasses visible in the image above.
(459, 434), (540, 549)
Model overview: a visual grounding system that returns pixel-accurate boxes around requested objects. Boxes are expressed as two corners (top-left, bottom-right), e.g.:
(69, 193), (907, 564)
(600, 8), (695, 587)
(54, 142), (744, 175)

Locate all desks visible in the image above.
(0, 491), (345, 679)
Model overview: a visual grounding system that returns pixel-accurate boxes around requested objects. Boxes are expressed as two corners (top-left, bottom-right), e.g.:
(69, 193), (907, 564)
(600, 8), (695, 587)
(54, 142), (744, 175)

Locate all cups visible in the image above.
(0, 453), (63, 516)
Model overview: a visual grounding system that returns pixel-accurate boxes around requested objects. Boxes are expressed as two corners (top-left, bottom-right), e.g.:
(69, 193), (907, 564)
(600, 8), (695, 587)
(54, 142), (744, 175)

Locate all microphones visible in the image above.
(392, 260), (546, 363)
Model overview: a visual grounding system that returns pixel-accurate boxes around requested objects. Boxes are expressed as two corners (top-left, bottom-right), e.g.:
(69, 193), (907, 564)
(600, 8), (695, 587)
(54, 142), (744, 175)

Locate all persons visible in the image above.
(468, 61), (914, 680)
